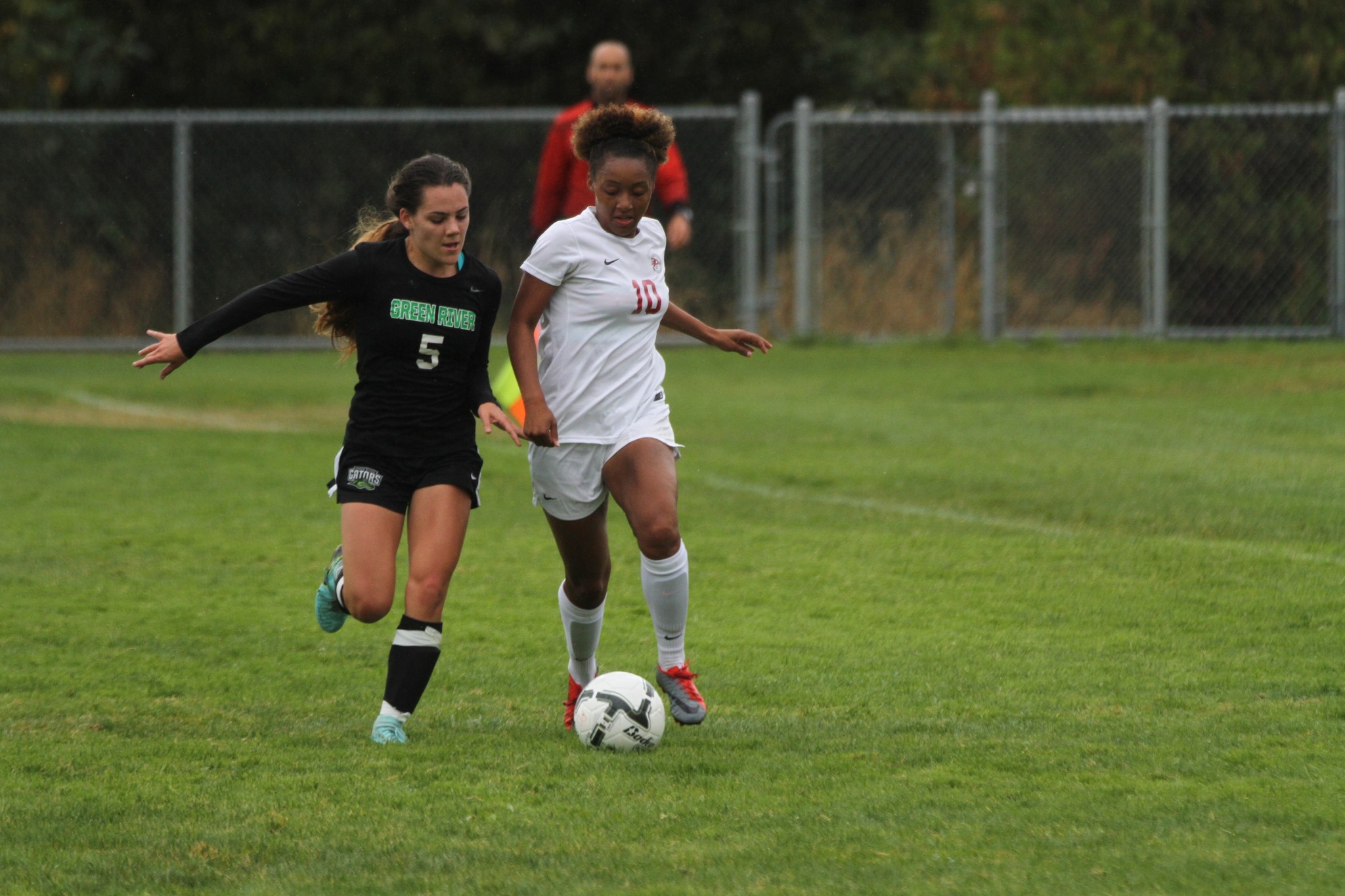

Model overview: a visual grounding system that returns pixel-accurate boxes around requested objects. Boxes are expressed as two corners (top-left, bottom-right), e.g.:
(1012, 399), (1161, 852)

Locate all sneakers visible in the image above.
(314, 545), (348, 635)
(562, 665), (599, 732)
(654, 659), (707, 727)
(370, 715), (407, 746)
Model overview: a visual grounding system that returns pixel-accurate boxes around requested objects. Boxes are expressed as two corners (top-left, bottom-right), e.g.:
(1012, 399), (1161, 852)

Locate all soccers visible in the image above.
(573, 671), (666, 750)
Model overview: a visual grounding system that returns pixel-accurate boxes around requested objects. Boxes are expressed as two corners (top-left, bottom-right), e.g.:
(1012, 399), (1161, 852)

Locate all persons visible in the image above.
(530, 38), (693, 252)
(506, 103), (774, 731)
(130, 153), (532, 745)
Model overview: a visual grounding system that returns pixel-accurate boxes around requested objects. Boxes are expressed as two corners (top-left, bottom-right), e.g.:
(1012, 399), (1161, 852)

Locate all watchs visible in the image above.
(671, 206), (693, 221)
